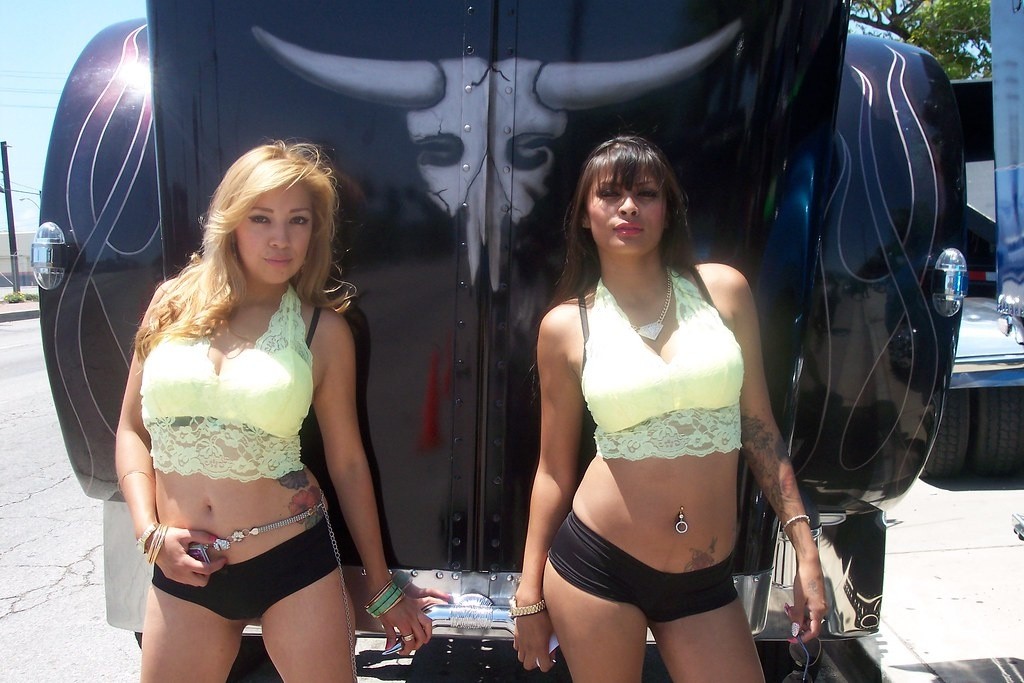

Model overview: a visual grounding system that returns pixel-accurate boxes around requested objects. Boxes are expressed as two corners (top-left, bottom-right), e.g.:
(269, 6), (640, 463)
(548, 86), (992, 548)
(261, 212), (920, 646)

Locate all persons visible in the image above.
(115, 144), (433, 683)
(342, 565), (452, 633)
(513, 136), (825, 682)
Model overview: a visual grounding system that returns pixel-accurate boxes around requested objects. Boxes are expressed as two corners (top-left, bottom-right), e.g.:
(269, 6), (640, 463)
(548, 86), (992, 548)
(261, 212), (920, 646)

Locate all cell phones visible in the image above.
(187, 543), (211, 564)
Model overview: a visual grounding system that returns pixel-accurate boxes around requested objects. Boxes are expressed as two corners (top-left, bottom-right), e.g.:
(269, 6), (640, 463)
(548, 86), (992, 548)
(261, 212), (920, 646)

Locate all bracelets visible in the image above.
(363, 580), (405, 618)
(147, 524), (168, 566)
(783, 514), (810, 534)
(392, 569), (412, 590)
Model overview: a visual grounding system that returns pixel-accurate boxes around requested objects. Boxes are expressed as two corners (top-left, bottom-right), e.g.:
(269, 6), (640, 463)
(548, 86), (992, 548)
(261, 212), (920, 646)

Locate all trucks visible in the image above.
(919, 77), (1023, 483)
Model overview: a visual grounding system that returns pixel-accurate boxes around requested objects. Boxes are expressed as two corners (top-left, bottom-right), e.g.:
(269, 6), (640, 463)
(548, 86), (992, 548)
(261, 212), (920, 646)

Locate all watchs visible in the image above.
(509, 597), (545, 619)
(136, 523), (159, 555)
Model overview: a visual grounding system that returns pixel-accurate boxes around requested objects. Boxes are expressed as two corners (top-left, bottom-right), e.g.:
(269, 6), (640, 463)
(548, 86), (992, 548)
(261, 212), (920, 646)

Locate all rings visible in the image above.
(821, 618), (825, 624)
(403, 633), (414, 641)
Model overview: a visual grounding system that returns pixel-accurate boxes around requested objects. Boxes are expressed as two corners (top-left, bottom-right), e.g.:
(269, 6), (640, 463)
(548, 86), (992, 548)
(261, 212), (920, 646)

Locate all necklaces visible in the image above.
(611, 263), (672, 340)
